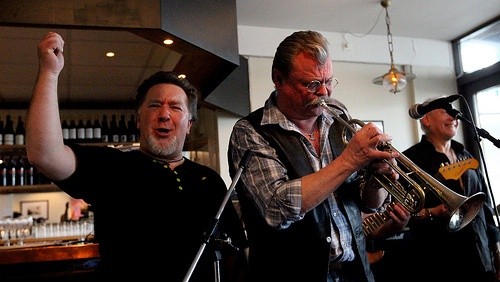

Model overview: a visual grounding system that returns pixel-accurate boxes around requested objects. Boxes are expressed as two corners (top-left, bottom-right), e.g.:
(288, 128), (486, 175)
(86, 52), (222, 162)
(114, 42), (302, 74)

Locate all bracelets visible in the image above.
(425, 207), (433, 221)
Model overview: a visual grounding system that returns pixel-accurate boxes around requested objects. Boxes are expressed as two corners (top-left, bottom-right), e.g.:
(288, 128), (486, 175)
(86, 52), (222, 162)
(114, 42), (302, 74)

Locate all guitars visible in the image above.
(356, 156), (479, 261)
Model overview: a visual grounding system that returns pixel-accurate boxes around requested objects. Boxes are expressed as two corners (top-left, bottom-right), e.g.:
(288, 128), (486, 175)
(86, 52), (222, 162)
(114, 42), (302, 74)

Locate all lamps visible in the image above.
(371, 0), (417, 94)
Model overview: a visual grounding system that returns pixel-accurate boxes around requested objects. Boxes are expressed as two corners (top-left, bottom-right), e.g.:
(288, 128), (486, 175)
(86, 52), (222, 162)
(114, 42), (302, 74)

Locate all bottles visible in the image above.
(0, 154), (37, 186)
(0, 113), (25, 146)
(59, 112), (140, 144)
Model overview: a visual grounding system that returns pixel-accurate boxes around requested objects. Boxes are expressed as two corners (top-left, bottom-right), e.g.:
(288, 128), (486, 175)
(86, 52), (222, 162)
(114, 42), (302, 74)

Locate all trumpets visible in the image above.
(310, 95), (487, 232)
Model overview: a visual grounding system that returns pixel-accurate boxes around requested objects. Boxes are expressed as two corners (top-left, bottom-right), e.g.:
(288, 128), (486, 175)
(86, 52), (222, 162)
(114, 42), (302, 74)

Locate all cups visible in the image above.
(0, 216), (95, 247)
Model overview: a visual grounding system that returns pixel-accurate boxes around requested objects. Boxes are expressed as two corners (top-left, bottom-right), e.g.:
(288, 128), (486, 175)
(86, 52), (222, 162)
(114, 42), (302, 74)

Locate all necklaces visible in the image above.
(166, 157), (184, 164)
(306, 124), (317, 142)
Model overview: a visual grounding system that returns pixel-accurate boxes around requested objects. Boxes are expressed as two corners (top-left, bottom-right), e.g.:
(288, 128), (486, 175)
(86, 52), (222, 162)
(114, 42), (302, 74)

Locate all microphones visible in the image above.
(409, 94), (461, 119)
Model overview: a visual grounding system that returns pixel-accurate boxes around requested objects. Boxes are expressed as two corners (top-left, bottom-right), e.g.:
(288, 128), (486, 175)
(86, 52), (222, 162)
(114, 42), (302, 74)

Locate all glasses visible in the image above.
(285, 76), (339, 93)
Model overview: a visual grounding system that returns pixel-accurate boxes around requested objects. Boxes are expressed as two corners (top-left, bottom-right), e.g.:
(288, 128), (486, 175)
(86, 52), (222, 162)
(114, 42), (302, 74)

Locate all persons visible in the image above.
(390, 98), (500, 282)
(23, 31), (250, 282)
(228, 31), (399, 282)
(361, 202), (411, 282)
